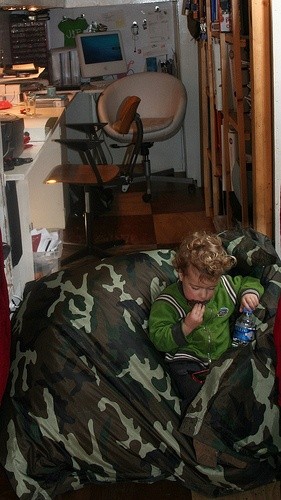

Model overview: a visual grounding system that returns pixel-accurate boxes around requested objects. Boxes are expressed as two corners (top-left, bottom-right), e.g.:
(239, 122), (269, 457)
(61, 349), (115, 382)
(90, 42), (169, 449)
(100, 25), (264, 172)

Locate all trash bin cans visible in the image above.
(29, 229), (63, 280)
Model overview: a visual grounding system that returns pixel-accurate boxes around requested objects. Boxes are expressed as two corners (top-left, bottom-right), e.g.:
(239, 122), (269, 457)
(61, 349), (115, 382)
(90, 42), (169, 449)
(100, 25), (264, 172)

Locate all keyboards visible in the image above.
(90, 80), (113, 88)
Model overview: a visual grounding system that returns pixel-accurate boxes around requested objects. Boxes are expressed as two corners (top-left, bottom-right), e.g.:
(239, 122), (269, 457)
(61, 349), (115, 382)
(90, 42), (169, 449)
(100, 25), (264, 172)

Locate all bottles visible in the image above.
(220, 8), (231, 33)
(231, 307), (256, 348)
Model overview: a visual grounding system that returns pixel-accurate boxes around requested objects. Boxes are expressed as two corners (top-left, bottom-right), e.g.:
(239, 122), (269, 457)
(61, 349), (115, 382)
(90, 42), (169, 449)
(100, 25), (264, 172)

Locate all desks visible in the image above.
(0, 81), (102, 313)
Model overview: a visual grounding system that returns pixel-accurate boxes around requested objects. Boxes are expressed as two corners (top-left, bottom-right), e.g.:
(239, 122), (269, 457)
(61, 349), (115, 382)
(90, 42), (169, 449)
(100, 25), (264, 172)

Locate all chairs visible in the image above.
(41, 95), (144, 268)
(99, 71), (197, 203)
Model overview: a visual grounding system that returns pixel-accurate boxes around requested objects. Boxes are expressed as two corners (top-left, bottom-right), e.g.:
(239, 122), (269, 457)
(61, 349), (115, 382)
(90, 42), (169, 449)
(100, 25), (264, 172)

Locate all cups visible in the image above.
(48, 87), (56, 97)
(23, 91), (37, 120)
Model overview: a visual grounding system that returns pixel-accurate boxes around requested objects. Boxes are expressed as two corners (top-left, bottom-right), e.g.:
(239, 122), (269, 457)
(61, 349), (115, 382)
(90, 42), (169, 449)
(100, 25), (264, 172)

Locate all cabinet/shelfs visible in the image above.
(198, 0), (276, 241)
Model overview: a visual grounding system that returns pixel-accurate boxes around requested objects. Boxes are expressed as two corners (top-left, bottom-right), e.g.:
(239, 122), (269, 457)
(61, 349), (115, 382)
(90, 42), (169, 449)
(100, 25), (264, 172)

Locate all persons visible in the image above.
(149, 231), (264, 467)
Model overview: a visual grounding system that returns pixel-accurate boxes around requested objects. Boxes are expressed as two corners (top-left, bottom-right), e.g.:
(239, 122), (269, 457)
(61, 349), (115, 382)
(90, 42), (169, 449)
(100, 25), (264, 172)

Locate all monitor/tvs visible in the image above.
(75, 30), (127, 83)
